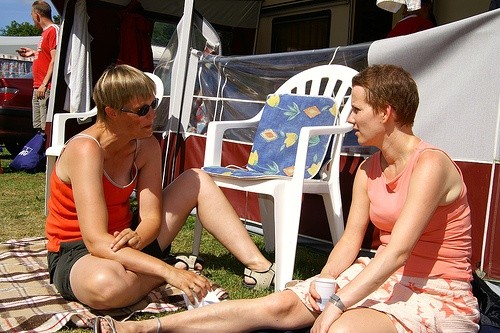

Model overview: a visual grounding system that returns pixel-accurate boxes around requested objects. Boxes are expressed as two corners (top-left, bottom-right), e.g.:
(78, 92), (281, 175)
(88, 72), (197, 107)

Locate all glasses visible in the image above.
(119, 98), (159, 116)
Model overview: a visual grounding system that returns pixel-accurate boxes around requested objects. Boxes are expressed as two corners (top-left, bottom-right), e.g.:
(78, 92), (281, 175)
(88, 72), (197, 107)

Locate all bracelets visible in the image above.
(329, 298), (344, 313)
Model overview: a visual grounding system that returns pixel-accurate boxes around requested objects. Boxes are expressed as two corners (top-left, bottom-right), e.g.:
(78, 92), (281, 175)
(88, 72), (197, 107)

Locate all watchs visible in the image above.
(330, 294), (346, 311)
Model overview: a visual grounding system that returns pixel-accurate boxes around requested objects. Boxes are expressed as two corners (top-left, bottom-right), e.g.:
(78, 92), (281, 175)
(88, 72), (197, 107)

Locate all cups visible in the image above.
(315, 278), (336, 311)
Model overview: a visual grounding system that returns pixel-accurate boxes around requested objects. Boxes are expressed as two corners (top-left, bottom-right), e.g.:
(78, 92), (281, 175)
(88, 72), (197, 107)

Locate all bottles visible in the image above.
(0, 62), (25, 78)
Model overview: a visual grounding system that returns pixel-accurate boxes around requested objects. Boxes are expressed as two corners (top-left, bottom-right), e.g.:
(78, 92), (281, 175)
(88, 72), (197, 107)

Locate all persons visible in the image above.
(17, 0), (60, 134)
(94, 64), (480, 333)
(45, 65), (276, 310)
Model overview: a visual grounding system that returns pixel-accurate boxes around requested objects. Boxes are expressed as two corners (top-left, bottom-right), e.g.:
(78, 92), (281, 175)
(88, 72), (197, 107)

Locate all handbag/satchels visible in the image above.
(8, 131), (46, 173)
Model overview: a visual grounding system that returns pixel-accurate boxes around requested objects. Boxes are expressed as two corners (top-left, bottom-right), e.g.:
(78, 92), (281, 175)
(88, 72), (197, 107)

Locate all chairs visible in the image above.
(192, 65), (360, 292)
(45, 72), (164, 216)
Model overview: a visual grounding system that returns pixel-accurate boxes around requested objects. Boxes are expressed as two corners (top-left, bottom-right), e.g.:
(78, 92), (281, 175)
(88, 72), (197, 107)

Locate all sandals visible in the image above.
(94, 314), (162, 333)
(242, 263), (275, 290)
(163, 254), (206, 272)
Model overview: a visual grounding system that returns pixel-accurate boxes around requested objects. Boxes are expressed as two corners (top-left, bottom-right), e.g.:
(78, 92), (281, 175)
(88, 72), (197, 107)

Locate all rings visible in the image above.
(192, 285), (196, 290)
